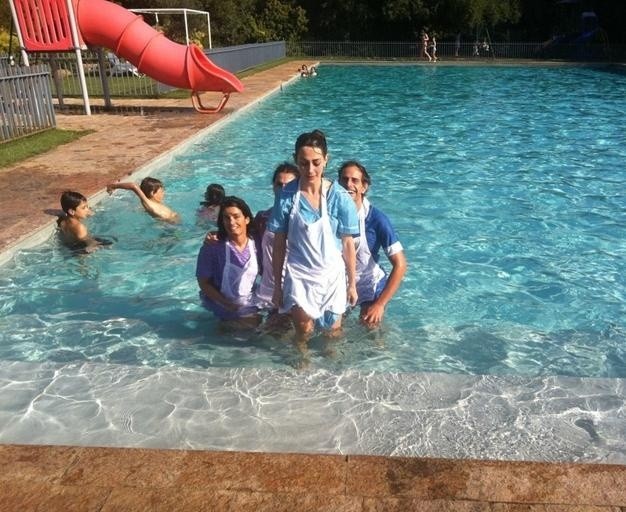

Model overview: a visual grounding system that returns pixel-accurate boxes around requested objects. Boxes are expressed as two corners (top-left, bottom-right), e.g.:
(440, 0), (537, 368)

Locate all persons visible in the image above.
(419, 31), (490, 63)
(298, 65), (318, 78)
(106, 177), (176, 224)
(59, 190), (96, 239)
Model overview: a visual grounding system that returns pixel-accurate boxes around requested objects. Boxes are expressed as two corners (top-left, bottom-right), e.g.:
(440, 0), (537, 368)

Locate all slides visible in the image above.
(72, 0), (244, 113)
